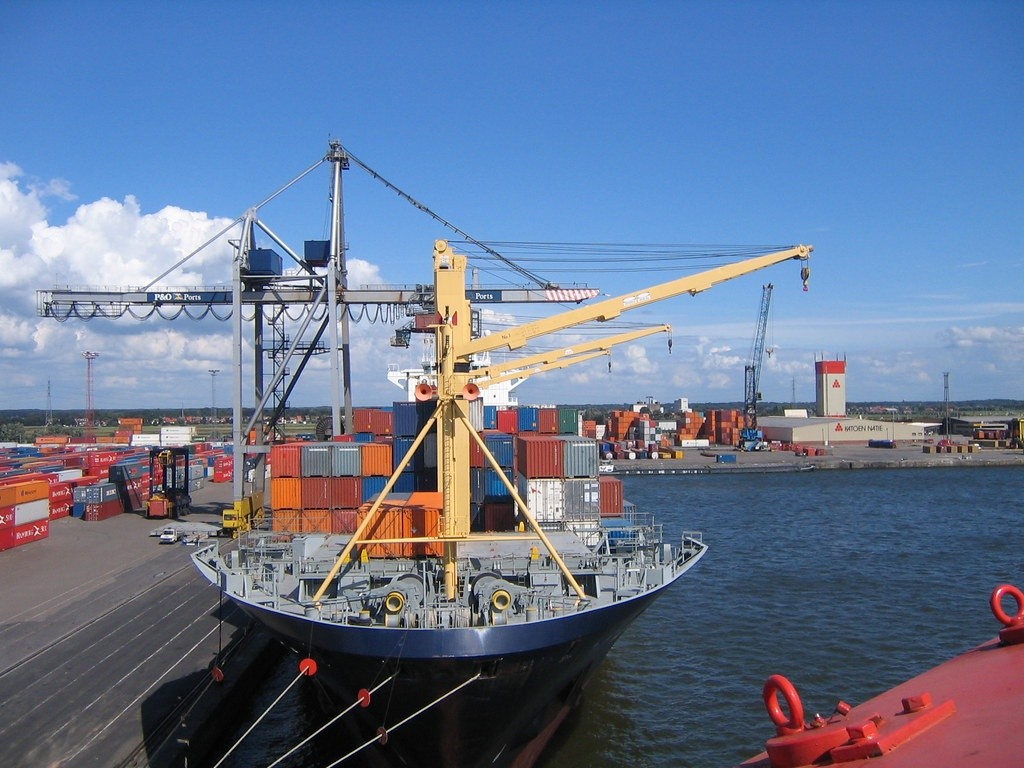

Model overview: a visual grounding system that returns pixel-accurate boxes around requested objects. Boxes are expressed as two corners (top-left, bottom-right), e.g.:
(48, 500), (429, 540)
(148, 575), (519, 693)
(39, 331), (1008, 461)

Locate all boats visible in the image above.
(186, 401), (712, 768)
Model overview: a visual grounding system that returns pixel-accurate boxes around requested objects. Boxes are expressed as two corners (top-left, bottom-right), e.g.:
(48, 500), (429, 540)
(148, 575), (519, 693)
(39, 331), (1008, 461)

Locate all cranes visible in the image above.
(32, 138), (814, 631)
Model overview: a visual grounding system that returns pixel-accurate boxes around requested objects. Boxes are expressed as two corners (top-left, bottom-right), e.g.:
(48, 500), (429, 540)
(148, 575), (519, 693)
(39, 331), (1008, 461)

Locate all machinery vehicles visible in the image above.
(738, 282), (774, 453)
(144, 446), (192, 520)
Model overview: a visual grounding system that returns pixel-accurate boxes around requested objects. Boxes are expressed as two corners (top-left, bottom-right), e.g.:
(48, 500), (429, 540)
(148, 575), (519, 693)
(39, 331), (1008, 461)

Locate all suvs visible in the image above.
(159, 527), (178, 544)
